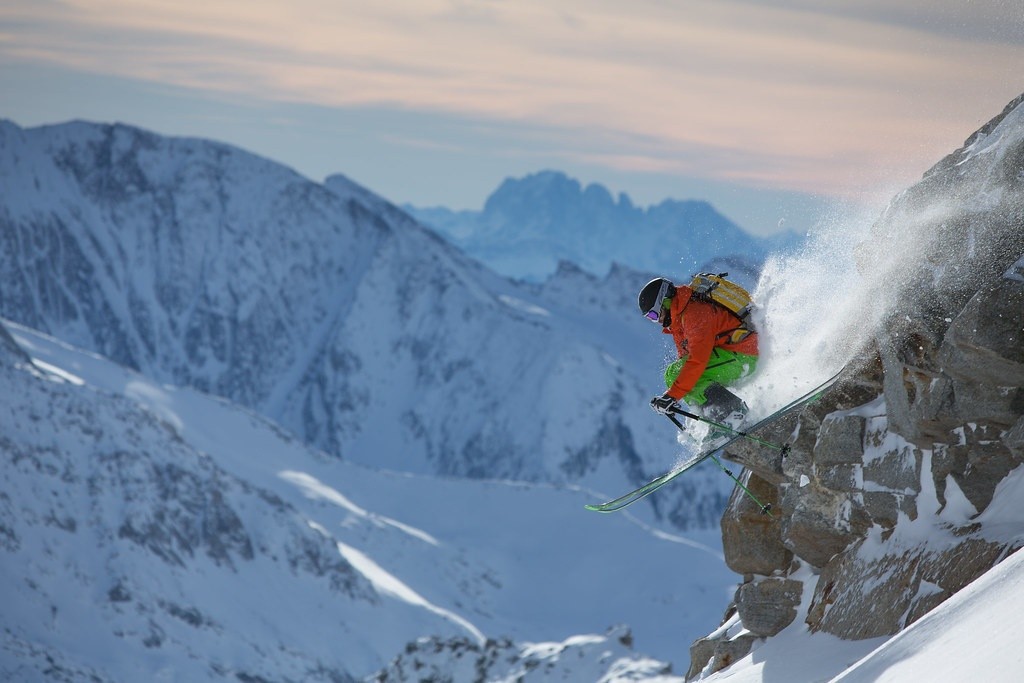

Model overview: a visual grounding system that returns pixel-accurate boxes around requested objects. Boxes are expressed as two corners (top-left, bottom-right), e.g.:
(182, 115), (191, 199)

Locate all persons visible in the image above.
(638, 278), (759, 443)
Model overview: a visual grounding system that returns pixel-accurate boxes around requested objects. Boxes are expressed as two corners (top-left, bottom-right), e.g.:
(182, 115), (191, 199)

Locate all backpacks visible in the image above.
(688, 271), (759, 324)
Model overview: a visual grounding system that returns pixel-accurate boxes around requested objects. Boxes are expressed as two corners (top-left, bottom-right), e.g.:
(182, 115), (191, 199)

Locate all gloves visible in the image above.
(650, 395), (682, 417)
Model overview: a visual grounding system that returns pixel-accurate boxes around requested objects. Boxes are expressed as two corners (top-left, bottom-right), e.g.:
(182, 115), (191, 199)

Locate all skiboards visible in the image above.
(582, 358), (854, 514)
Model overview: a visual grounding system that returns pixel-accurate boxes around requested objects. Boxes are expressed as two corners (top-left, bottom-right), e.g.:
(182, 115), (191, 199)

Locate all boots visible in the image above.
(702, 382), (749, 444)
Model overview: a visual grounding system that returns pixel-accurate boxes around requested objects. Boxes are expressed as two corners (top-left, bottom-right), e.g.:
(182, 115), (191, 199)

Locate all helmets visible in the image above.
(638, 278), (676, 315)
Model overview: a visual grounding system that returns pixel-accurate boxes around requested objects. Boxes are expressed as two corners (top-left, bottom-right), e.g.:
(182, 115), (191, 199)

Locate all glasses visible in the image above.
(644, 308), (661, 323)
